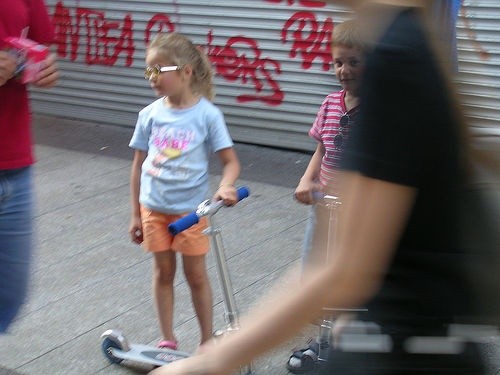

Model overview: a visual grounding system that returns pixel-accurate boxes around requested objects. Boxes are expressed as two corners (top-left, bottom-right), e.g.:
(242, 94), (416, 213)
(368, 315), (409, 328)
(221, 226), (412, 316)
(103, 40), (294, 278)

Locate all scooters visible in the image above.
(99, 183), (253, 374)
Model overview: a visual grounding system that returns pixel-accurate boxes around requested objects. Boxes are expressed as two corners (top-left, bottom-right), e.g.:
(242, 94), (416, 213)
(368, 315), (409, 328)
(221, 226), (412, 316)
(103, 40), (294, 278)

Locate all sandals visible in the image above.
(286, 336), (320, 374)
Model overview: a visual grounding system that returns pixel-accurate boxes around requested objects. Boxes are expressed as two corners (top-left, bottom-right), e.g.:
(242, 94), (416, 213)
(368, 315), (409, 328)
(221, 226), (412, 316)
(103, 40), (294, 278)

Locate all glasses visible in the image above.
(333, 112), (351, 152)
(143, 64), (185, 80)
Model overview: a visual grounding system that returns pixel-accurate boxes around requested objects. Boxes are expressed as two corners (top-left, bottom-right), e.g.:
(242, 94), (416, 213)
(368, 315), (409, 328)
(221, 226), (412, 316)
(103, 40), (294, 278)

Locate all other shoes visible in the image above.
(156, 339), (178, 351)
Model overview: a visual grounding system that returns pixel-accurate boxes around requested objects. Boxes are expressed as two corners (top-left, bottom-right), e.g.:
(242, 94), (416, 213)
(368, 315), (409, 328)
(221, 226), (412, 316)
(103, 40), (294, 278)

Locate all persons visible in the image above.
(129, 32), (242, 354)
(287, 19), (370, 372)
(147, 1), (500, 375)
(0, 1), (57, 338)
